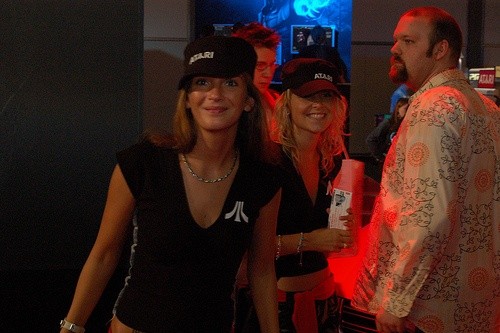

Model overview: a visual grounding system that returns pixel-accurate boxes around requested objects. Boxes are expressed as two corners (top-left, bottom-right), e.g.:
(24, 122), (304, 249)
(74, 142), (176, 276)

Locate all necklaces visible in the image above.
(179, 153), (239, 183)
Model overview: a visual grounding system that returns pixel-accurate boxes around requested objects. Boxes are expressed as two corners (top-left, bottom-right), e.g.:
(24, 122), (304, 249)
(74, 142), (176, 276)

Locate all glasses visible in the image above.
(256, 61), (278, 70)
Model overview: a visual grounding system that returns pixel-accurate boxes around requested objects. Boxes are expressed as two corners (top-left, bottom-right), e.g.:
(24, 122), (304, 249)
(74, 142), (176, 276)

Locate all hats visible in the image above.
(281, 57), (342, 97)
(183, 37), (258, 77)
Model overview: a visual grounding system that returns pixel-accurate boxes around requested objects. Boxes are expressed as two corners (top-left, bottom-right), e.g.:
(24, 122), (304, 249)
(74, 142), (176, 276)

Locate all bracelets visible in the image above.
(297, 232), (304, 254)
(60, 319), (85, 333)
(275, 234), (281, 260)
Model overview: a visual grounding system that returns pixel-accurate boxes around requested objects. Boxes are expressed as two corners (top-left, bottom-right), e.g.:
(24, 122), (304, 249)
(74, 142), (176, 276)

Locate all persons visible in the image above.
(350, 6), (500, 333)
(59, 34), (284, 333)
(231, 22), (284, 147)
(236, 55), (358, 333)
(298, 26), (346, 83)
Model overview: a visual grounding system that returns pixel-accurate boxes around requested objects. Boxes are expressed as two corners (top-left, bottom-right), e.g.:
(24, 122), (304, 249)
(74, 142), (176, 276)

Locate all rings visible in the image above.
(344, 243), (347, 248)
